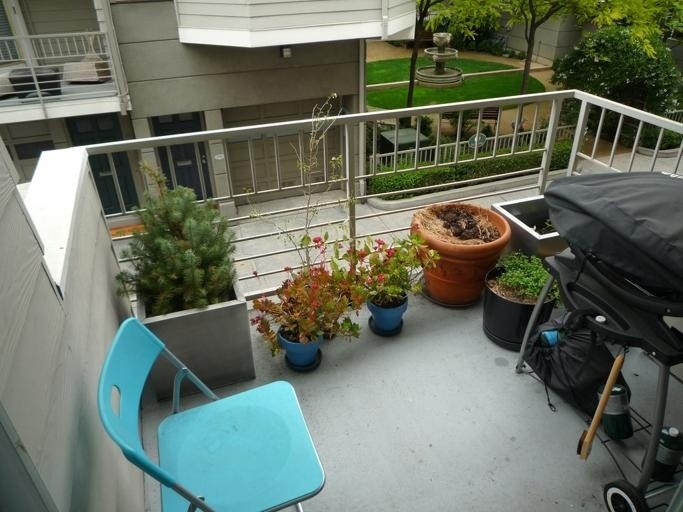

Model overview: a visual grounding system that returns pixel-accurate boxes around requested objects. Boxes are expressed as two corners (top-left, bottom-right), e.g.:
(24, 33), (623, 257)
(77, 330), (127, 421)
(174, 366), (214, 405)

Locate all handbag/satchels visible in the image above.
(524, 311), (630, 420)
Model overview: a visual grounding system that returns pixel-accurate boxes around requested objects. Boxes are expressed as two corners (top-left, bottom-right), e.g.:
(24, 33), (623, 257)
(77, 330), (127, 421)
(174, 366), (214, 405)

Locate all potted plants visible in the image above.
(113, 159), (256, 402)
(482, 249), (565, 352)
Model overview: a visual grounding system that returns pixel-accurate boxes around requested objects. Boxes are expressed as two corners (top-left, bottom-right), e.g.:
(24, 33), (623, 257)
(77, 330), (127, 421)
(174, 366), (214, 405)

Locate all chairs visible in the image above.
(97, 318), (325, 512)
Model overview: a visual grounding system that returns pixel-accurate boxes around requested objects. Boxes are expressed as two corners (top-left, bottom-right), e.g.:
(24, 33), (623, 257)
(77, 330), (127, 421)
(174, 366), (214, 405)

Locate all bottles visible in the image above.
(642, 426), (681, 482)
(596, 383), (633, 440)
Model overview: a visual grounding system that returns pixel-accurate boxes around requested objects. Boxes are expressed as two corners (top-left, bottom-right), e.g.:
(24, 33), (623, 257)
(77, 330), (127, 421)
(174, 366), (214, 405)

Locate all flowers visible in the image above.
(250, 225), (440, 357)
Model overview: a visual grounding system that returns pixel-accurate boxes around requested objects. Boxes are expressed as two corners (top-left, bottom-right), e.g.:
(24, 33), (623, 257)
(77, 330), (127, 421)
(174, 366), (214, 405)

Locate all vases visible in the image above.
(278, 292), (408, 372)
(411, 201), (511, 310)
